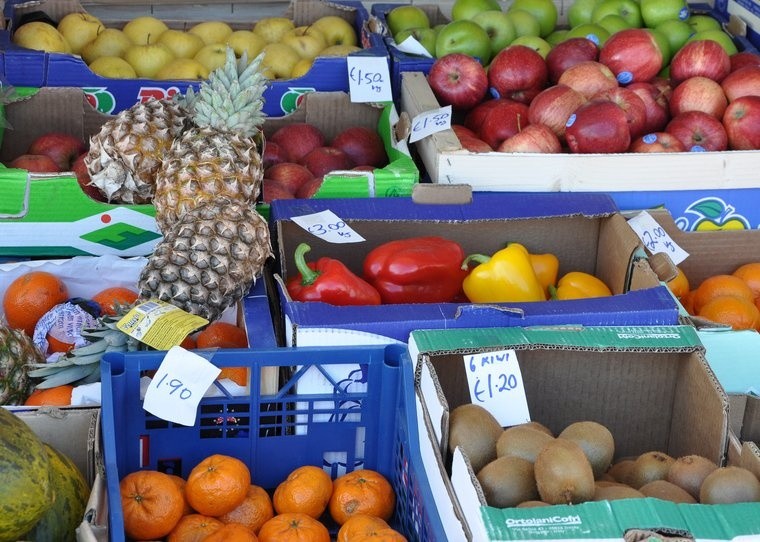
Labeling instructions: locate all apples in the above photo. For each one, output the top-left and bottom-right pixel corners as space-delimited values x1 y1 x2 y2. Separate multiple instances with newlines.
386 0 760 153
260 120 383 203
7 134 108 203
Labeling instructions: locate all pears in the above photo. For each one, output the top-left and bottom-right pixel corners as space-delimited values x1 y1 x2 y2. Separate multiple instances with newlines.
15 12 363 81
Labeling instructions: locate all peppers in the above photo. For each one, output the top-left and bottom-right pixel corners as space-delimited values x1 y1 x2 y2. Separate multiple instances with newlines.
286 236 614 306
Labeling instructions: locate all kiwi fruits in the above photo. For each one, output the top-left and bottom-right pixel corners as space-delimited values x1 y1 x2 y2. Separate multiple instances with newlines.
443 403 760 507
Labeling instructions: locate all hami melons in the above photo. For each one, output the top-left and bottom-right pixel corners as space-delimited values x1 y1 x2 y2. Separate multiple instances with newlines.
0 407 93 542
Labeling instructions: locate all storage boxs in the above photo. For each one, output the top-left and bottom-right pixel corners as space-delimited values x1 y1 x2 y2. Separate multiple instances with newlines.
0 0 760 542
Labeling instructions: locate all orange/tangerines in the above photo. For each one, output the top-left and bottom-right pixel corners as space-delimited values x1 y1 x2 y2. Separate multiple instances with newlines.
122 453 408 542
4 270 249 407
662 262 759 334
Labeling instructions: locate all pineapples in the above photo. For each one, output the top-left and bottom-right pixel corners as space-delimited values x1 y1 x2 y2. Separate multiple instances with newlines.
0 318 42 406
84 46 273 331
